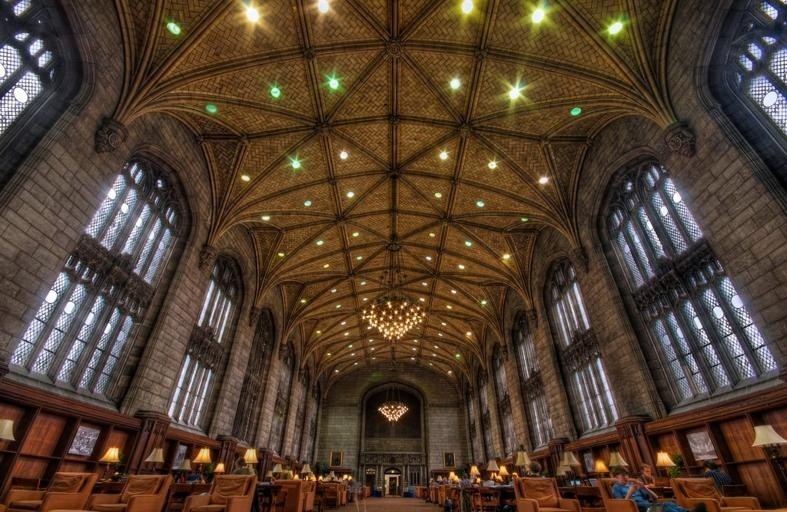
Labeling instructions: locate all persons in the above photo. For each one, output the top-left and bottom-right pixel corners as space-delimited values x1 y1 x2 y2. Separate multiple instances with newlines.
233 456 250 475
501 463 517 484
202 472 208 484
612 465 708 512
203 464 214 483
429 473 472 488
701 459 732 486
635 464 657 489
270 470 357 505
390 479 396 496
497 461 546 512
479 471 494 500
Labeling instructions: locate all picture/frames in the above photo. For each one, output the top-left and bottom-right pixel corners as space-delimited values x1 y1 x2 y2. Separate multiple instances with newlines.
443 450 456 469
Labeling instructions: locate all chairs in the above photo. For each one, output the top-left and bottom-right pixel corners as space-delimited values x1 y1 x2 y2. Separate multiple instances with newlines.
414 473 760 511
0 471 371 512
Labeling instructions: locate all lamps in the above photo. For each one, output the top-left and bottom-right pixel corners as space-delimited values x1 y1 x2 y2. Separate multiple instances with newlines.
0 416 19 442
750 424 787 480
377 342 410 423
360 232 426 340
468 441 629 481
655 450 677 479
99 448 120 479
142 446 352 483
